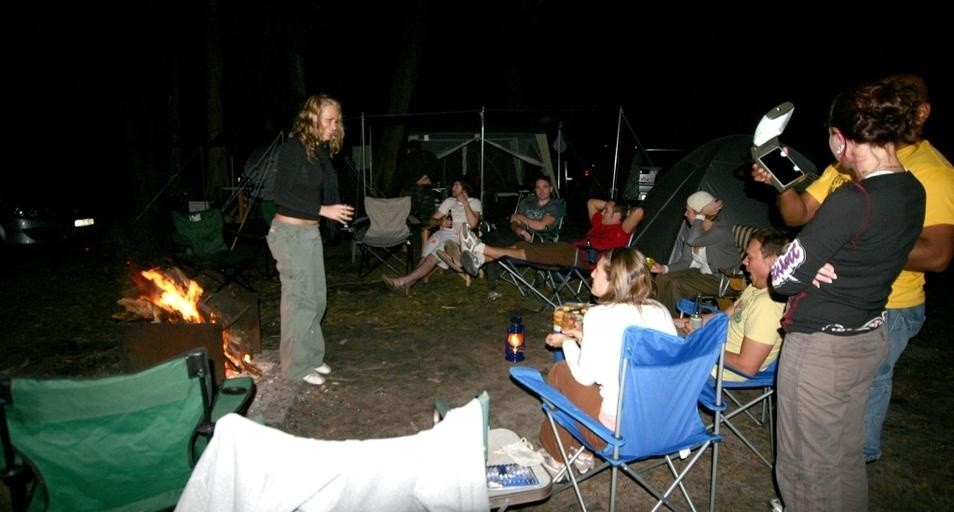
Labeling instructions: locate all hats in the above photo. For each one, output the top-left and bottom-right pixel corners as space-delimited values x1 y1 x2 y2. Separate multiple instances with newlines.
687 191 714 212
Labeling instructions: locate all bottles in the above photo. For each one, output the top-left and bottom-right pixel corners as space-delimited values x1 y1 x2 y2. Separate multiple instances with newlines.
585 237 592 248
447 208 453 231
690 291 706 336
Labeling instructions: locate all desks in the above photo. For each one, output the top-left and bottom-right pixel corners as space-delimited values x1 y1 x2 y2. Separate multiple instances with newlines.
484 426 555 512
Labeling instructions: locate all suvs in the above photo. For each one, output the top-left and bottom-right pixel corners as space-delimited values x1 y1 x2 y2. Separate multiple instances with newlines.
637 148 693 203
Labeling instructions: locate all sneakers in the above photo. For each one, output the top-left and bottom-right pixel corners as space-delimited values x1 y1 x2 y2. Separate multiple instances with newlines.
538 447 594 484
437 222 482 277
303 362 331 385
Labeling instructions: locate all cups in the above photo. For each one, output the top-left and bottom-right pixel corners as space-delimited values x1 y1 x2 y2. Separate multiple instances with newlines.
338 204 356 230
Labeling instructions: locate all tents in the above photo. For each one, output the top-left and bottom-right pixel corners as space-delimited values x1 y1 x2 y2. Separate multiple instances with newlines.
629 134 822 280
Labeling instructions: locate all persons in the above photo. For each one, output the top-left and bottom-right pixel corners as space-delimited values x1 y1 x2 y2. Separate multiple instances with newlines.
672 226 790 384
765 83 928 512
646 187 749 320
262 92 356 388
534 244 681 485
749 67 954 468
457 198 646 279
434 174 565 276
397 139 441 227
379 177 484 294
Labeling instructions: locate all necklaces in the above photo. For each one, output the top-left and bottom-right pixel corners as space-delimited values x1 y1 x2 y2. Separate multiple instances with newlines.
857 164 904 180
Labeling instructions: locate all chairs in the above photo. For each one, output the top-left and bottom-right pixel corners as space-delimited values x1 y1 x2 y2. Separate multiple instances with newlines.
530 225 637 309
667 297 786 470
169 384 495 512
507 310 726 512
498 196 565 296
168 206 256 296
348 186 488 296
0 341 261 512
648 222 770 320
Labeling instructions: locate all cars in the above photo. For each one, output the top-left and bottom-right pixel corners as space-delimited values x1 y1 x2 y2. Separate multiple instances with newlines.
1 171 105 259
